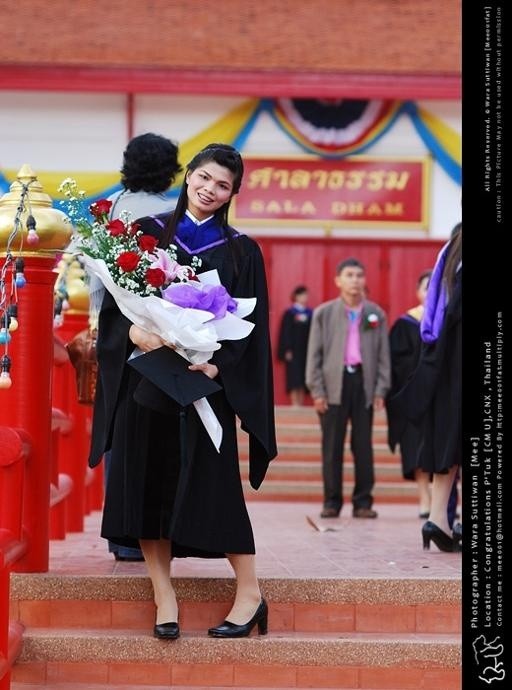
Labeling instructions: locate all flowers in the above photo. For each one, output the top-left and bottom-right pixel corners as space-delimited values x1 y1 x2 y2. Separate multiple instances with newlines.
60 179 256 367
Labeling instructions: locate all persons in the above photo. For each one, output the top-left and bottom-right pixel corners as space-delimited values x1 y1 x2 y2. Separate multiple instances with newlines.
77 129 463 640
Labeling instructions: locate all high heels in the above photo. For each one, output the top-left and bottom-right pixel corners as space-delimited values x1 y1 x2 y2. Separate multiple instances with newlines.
155 606 180 640
418 493 431 519
422 521 461 551
208 598 269 638
453 519 463 553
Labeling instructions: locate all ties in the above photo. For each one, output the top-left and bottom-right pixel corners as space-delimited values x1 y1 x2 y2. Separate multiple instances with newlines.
351 311 359 320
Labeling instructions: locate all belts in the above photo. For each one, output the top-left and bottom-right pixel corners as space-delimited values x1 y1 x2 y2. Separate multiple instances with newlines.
345 364 360 372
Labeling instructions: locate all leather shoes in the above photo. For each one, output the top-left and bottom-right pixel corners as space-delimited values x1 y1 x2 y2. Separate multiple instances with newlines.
320 508 341 518
351 508 378 518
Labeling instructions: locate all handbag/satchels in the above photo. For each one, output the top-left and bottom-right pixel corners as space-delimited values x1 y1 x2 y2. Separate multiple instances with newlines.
65 329 98 402
